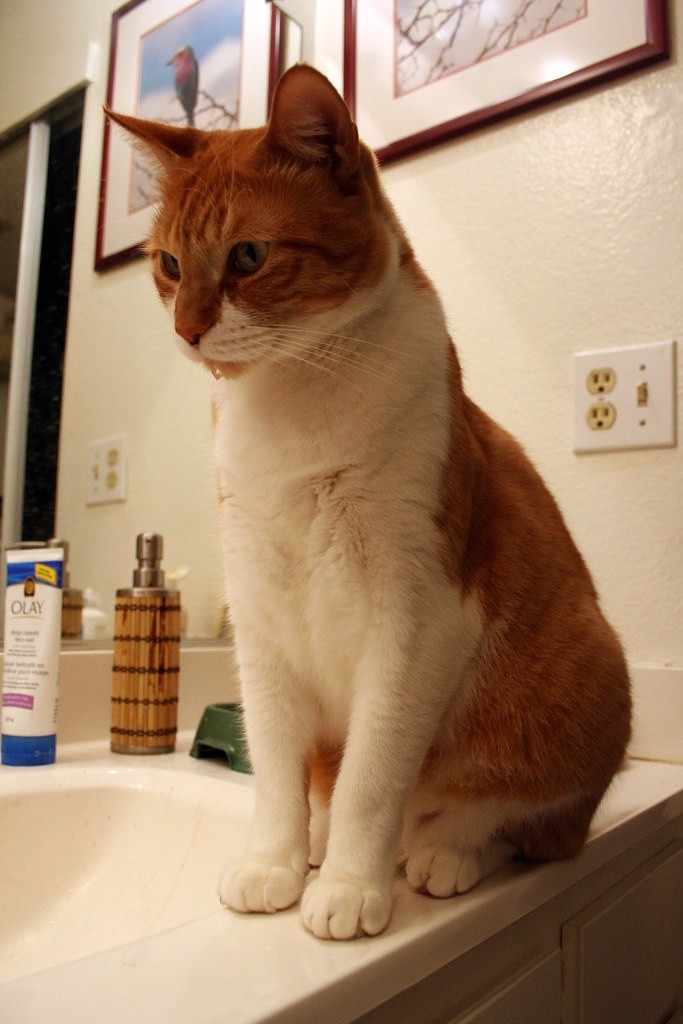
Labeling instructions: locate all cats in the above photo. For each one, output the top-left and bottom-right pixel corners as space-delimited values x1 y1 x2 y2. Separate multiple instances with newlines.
102 61 632 942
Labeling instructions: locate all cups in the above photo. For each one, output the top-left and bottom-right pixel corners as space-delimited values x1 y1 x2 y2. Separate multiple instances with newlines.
187 599 225 640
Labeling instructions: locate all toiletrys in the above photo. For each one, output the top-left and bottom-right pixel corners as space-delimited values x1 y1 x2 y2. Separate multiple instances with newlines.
107 531 183 757
0 547 65 768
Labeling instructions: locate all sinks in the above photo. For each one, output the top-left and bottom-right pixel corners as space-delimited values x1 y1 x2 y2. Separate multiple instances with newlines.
0 751 323 986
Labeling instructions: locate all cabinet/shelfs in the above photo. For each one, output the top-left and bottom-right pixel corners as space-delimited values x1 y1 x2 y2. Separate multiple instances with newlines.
348 811 683 1024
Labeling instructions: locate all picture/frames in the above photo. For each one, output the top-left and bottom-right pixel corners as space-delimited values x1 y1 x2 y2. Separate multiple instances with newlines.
92 0 281 270
342 0 673 169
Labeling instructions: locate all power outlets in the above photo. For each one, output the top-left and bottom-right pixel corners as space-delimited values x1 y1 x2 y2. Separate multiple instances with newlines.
572 338 674 453
85 434 128 506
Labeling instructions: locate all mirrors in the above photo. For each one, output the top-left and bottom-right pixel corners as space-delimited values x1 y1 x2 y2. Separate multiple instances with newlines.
0 0 352 648
